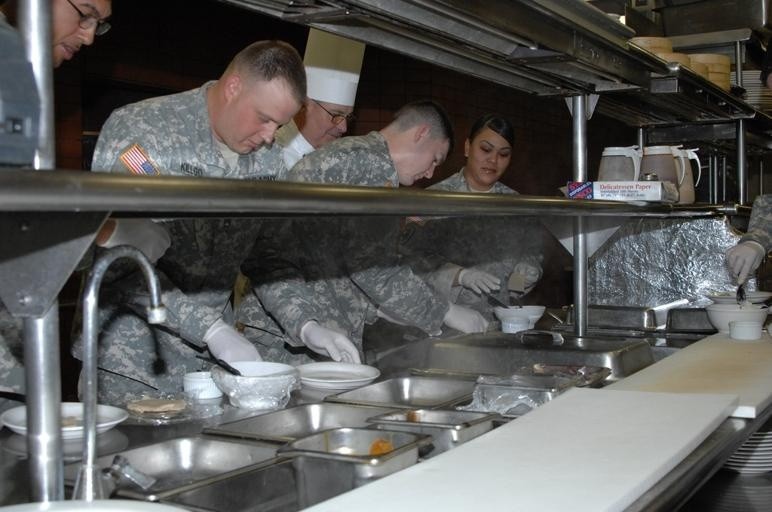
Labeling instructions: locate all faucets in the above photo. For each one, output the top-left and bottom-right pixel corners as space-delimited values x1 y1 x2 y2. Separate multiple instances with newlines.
72 241 168 504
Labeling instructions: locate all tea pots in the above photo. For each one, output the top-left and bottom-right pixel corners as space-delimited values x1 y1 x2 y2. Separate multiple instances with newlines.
597 144 640 182
642 143 686 186
672 147 702 206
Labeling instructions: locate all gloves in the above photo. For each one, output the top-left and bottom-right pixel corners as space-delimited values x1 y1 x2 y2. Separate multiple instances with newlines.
443 301 488 333
458 267 500 294
300 320 361 364
97 219 171 264
514 263 539 288
725 241 765 285
377 310 442 337
202 318 262 361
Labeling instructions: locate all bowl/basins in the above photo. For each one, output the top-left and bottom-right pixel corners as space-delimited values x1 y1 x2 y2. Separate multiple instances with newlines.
493 304 546 331
211 360 301 410
501 318 530 334
704 304 769 335
183 372 225 400
728 321 763 341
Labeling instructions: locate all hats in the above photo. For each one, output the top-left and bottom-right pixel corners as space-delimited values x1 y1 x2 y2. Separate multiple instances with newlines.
303 28 365 107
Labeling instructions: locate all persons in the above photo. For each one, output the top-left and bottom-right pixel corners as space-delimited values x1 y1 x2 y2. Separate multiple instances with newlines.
0 1 173 393
401 111 553 326
268 96 357 175
70 39 362 412
724 193 772 286
234 98 490 365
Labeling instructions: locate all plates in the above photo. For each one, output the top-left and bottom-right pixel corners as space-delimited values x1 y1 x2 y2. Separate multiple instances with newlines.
0 428 129 461
293 361 382 391
710 292 772 304
730 69 772 112
722 427 772 475
714 477 772 512
0 399 131 441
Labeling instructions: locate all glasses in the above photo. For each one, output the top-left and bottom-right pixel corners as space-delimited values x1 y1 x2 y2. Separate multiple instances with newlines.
314 102 359 124
68 0 111 36
